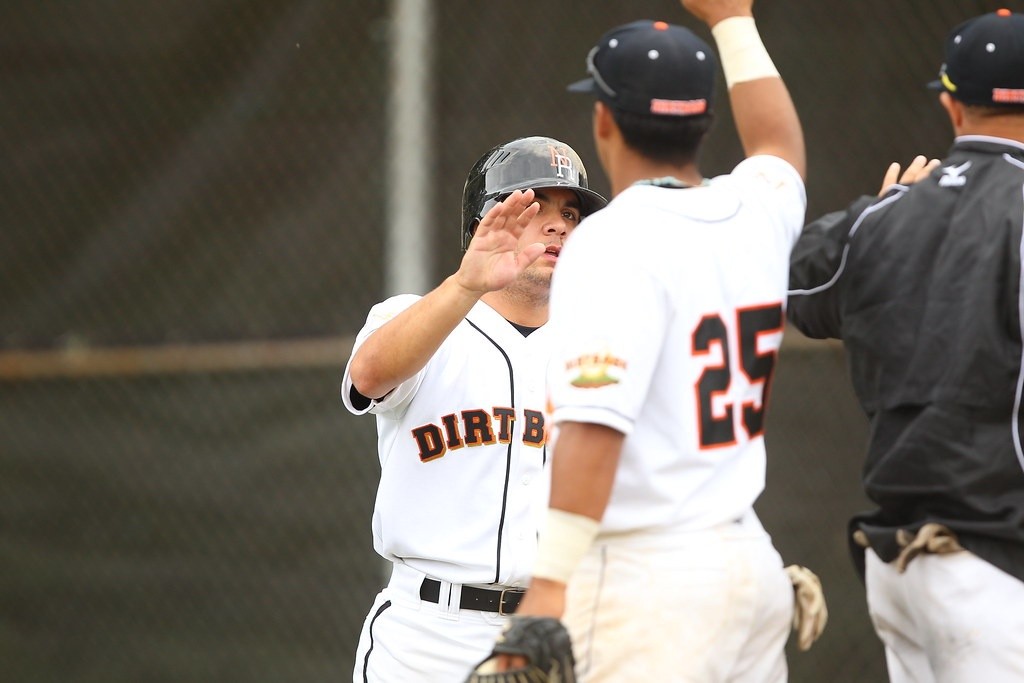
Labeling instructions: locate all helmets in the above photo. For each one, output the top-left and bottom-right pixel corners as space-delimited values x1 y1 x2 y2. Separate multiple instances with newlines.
461 135 607 252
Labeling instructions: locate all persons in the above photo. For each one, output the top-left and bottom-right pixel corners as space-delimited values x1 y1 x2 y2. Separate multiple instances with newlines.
786 9 1024 683
341 136 609 683
468 0 806 683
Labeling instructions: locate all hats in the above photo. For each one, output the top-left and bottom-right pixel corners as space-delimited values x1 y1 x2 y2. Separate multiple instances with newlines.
566 19 716 117
925 8 1024 107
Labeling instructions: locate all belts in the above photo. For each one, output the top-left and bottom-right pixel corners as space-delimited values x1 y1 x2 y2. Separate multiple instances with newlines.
419 577 525 616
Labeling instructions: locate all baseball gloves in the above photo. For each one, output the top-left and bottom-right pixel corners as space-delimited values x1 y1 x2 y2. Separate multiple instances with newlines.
466 615 577 683
